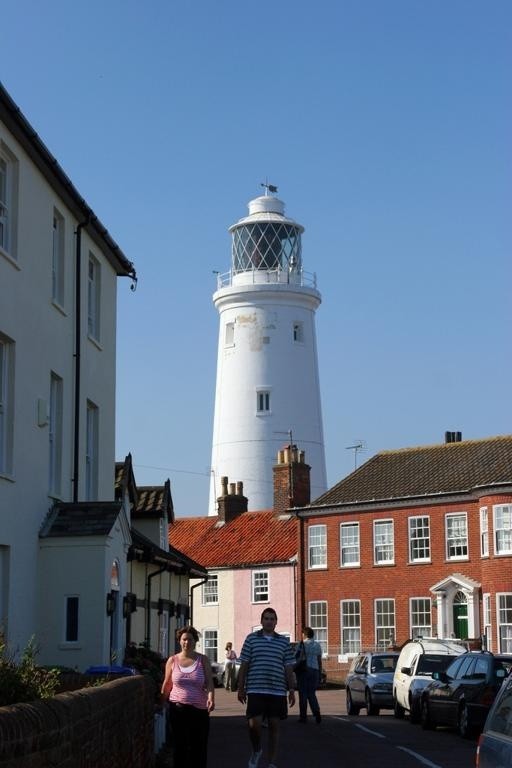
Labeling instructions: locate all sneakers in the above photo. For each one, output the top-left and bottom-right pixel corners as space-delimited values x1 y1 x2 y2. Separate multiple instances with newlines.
247 748 264 767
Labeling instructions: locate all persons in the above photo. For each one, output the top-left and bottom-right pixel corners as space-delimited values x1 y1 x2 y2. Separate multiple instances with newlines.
225 642 237 692
237 608 296 767
296 627 322 723
160 626 215 767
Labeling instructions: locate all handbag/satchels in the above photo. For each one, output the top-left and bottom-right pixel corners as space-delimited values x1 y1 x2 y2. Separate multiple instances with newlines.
294 640 307 663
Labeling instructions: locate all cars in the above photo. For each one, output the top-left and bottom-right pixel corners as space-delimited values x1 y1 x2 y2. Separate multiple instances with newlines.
345 636 512 768
211 660 326 690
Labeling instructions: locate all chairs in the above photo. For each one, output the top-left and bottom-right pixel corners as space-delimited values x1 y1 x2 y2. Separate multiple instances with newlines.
373 659 384 673
